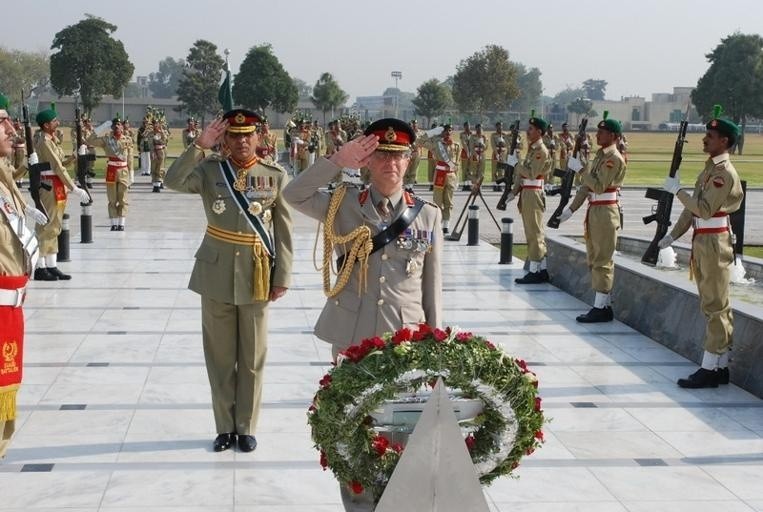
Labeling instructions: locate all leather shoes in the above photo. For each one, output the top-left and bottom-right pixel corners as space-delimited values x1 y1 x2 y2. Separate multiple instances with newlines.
111 225 124 231
238 435 256 452
678 367 729 388
576 305 613 322
34 267 71 281
213 433 236 452
515 268 549 284
152 187 160 192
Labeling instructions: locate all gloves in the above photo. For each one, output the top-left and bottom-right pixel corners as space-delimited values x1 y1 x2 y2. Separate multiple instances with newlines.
567 151 584 174
23 152 39 170
661 169 683 195
505 193 515 205
507 151 518 167
426 126 444 138
556 208 573 223
657 233 674 250
127 171 135 187
93 121 113 137
24 204 48 226
72 187 90 204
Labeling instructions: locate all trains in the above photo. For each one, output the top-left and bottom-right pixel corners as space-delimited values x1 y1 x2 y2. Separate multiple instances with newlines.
658 122 762 133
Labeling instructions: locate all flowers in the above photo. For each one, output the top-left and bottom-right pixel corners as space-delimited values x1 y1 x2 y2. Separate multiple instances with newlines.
305 321 546 502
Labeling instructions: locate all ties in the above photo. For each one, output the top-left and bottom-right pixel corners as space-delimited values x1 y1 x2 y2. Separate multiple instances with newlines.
382 198 389 213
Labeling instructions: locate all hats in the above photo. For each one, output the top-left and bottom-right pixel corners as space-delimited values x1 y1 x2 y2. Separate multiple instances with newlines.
363 118 417 153
0 92 9 111
529 118 549 131
598 119 622 134
113 117 121 123
706 118 740 137
222 109 264 134
36 109 58 126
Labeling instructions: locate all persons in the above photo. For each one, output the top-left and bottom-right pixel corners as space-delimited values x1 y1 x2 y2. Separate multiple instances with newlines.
73 108 96 191
182 117 200 148
557 118 626 323
658 116 734 390
32 107 90 282
10 118 27 190
502 119 552 286
88 118 136 232
164 110 294 453
404 120 638 235
280 117 444 364
0 90 25 458
258 113 373 172
136 104 171 193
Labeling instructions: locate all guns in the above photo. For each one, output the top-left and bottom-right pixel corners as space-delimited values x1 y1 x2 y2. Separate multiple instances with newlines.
76 96 95 206
496 120 520 210
641 103 691 266
546 118 588 228
22 90 52 226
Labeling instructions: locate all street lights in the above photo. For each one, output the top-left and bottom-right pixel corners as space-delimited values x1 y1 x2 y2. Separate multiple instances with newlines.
390 70 403 112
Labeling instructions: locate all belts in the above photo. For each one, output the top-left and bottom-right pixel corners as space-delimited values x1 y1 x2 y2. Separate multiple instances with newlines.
522 178 542 188
474 147 483 152
435 165 454 171
154 145 163 149
107 160 128 167
257 150 271 155
0 285 27 308
495 147 506 151
588 192 617 202
41 170 56 176
691 216 729 230
